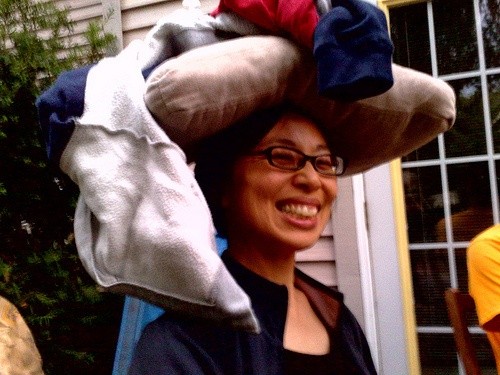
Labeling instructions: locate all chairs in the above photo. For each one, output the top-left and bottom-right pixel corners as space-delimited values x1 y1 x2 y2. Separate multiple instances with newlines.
446 289 500 375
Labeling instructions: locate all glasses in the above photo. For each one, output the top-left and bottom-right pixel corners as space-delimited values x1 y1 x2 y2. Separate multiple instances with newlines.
236 145 345 178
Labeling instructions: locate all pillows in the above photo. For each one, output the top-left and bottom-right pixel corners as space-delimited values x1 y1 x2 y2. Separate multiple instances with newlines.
142 35 457 178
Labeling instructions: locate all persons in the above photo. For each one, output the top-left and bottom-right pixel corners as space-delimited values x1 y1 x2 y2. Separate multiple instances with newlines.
126 103 381 375
437 167 500 326
